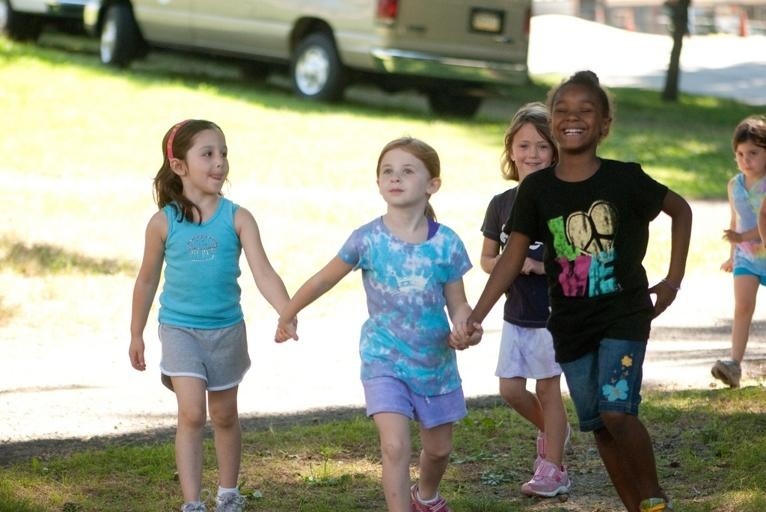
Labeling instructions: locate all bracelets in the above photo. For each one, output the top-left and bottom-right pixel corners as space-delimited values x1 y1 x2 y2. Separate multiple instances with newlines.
660 276 680 293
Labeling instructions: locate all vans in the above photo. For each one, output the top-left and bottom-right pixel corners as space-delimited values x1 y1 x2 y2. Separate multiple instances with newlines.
67 0 535 121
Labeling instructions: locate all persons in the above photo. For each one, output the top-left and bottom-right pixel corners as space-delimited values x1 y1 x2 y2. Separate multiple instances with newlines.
713 112 766 386
275 135 484 512
462 70 692 511
477 100 582 499
127 120 295 511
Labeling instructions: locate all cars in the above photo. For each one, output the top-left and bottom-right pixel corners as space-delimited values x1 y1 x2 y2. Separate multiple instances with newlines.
0 0 54 43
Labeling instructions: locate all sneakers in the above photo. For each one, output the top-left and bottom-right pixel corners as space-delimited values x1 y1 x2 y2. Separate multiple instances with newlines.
521 459 571 496
533 422 570 472
214 490 247 512
711 359 741 387
410 479 454 512
639 498 674 512
180 501 206 512
